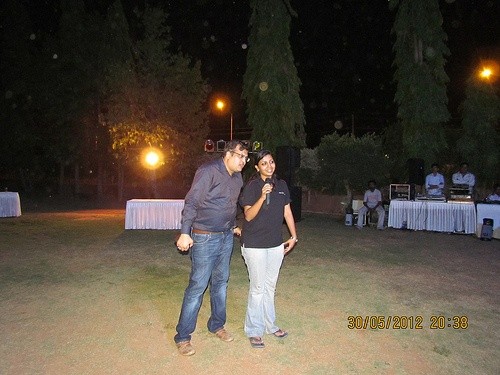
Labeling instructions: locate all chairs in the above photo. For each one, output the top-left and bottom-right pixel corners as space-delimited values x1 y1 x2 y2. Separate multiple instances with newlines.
352 200 366 225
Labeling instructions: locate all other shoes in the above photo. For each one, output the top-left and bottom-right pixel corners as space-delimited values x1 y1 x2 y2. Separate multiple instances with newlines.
214 327 234 342
176 341 196 356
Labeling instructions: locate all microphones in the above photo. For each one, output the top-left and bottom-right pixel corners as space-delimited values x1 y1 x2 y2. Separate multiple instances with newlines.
264 178 272 205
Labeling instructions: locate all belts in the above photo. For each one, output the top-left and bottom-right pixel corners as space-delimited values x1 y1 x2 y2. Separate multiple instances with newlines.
191 228 225 234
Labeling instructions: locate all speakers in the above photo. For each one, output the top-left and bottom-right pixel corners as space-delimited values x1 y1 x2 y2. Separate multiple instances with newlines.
275 145 301 169
408 158 425 185
283 186 301 223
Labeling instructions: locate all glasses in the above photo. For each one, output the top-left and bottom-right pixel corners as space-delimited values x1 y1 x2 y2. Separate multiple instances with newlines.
230 151 250 163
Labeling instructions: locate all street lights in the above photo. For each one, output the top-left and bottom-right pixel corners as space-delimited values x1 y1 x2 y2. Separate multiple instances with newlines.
217 101 233 141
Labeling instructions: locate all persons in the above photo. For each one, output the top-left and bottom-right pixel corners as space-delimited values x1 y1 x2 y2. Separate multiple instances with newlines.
425 164 444 196
174 142 250 355
453 163 476 195
485 184 500 201
239 150 298 348
355 181 386 231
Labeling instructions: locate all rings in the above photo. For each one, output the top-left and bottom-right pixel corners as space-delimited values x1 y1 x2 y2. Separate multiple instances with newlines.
184 248 187 249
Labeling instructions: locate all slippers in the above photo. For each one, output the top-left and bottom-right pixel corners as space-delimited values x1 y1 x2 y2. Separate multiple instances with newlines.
273 329 287 338
249 337 265 348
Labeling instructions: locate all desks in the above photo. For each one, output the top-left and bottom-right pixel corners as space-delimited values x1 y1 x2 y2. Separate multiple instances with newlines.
0 192 21 217
474 199 500 239
388 199 477 234
125 199 185 230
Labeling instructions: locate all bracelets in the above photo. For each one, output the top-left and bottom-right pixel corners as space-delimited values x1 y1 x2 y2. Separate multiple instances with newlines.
233 226 238 229
291 237 298 242
